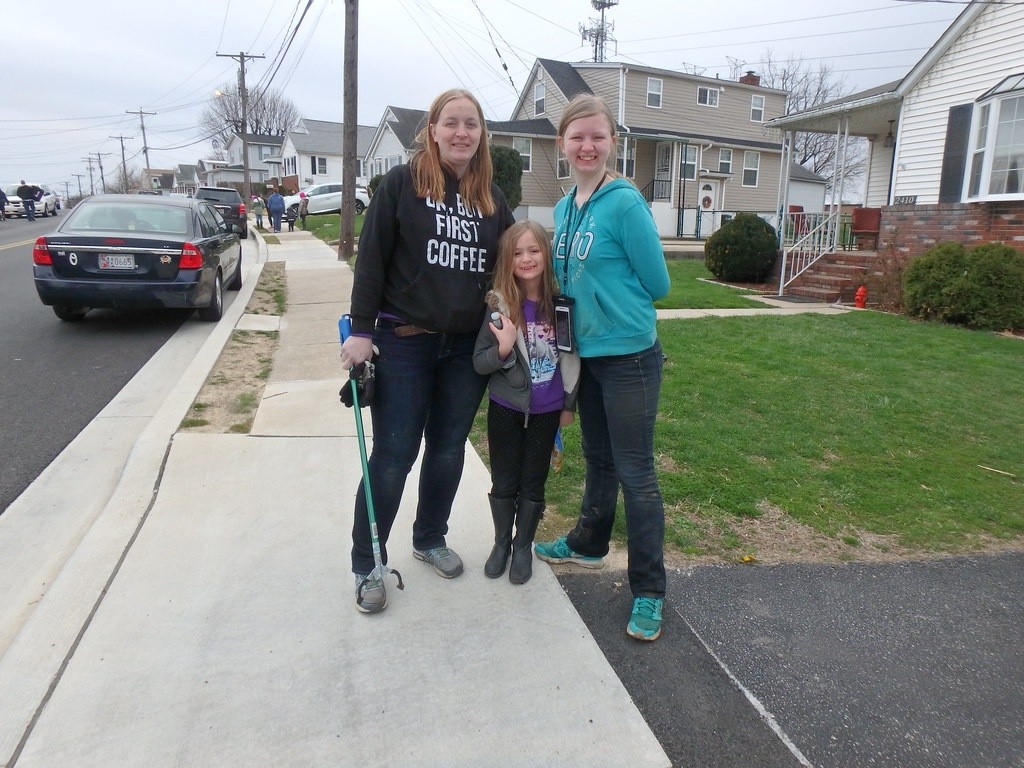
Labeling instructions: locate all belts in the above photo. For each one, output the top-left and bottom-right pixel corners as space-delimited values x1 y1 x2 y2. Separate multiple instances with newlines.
395 323 439 338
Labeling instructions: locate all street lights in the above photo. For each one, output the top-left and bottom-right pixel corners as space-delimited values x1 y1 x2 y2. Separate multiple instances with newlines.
215 90 251 213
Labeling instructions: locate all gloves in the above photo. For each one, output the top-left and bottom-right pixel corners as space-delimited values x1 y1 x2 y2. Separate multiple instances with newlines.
339 343 379 409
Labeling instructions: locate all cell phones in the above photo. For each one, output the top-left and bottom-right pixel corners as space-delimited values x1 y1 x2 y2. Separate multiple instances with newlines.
554 305 572 353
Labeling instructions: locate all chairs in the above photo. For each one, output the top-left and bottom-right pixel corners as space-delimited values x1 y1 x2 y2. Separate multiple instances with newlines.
787 205 833 254
848 207 881 253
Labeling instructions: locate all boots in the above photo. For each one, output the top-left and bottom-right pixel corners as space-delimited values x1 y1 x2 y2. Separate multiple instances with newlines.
257 221 259 228
483 493 516 579
260 221 263 229
509 496 546 584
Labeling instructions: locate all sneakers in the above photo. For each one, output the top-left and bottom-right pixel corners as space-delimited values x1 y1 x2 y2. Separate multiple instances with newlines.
534 535 606 569
413 545 463 579
626 598 665 642
354 573 388 613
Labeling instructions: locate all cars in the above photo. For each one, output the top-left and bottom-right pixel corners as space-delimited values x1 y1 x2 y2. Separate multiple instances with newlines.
4 184 61 218
33 194 244 321
282 183 370 218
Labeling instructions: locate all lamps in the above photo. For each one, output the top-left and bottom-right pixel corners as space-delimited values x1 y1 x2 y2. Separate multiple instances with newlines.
883 120 897 148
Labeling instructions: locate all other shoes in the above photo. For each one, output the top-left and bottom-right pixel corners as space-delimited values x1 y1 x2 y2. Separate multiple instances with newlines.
275 229 280 233
30 215 35 221
2 216 5 221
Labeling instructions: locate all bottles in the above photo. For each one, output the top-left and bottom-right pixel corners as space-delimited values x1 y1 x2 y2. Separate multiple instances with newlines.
490 311 517 369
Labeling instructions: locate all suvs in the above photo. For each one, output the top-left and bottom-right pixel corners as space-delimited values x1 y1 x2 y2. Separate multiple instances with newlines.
193 187 247 239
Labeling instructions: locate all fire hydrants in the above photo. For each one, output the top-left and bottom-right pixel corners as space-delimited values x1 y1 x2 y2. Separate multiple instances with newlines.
855 285 868 307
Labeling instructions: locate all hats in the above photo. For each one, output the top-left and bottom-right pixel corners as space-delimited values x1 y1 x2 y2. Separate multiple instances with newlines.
300 192 305 198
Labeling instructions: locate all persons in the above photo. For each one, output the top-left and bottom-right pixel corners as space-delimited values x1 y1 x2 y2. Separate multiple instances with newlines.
340 89 517 613
17 180 36 222
253 194 275 229
472 218 581 585
284 206 298 232
268 188 285 233
534 94 670 641
0 189 9 221
298 192 309 231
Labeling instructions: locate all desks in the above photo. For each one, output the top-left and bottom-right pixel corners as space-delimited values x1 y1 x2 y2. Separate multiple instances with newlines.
818 219 855 252
